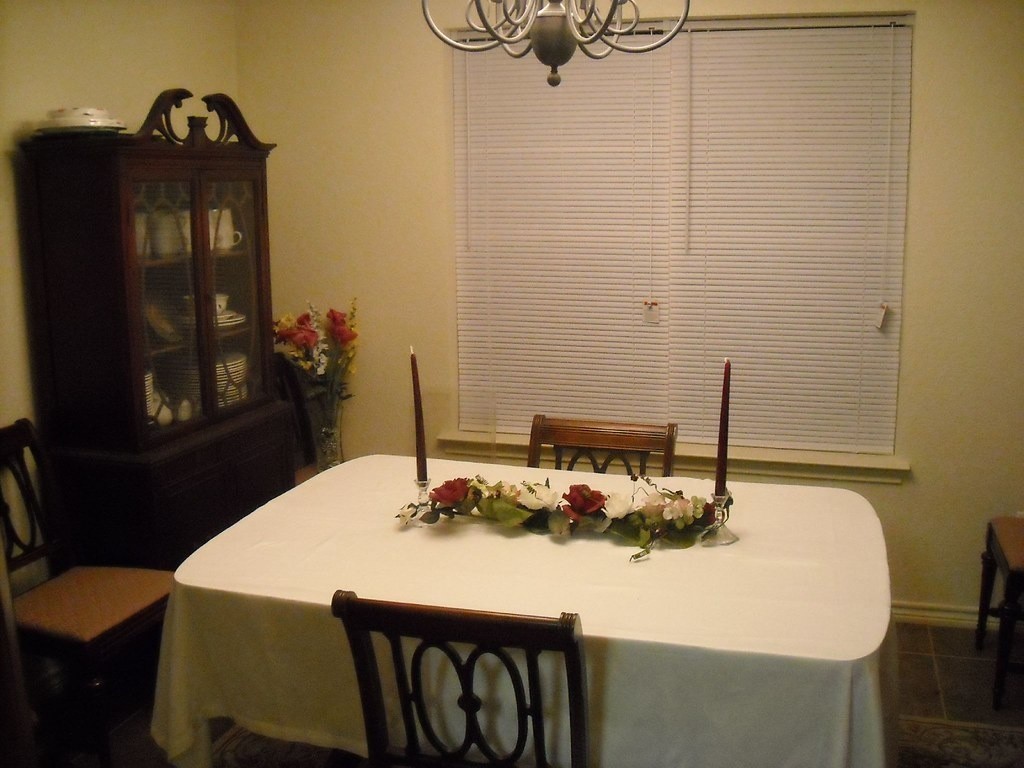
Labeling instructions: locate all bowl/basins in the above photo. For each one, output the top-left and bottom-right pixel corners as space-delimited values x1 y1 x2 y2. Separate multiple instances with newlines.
177 294 229 316
130 211 242 258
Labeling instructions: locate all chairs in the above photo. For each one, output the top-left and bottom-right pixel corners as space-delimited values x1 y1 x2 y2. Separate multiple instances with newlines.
0 418 174 768
331 589 589 768
527 414 678 477
975 516 1024 711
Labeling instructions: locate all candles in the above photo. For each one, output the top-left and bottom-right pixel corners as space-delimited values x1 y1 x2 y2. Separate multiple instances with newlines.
410 346 427 482
714 357 731 497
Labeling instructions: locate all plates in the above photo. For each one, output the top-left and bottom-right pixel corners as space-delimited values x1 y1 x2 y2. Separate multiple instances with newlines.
176 313 247 332
143 349 251 426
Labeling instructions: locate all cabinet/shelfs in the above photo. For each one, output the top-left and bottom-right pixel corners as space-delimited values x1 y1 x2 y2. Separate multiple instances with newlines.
19 88 295 570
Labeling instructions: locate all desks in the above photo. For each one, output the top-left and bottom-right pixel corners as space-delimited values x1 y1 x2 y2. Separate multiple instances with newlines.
150 453 900 768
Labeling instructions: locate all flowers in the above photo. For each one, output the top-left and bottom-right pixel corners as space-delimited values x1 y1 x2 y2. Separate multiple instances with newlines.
272 297 359 475
394 474 733 563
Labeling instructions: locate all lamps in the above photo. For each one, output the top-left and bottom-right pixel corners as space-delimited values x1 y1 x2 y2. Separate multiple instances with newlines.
421 0 690 87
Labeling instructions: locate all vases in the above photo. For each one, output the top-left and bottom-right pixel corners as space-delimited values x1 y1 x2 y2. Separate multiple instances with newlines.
301 379 342 471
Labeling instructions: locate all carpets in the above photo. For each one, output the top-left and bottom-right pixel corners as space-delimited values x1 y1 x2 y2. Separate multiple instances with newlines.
206 713 1024 768
